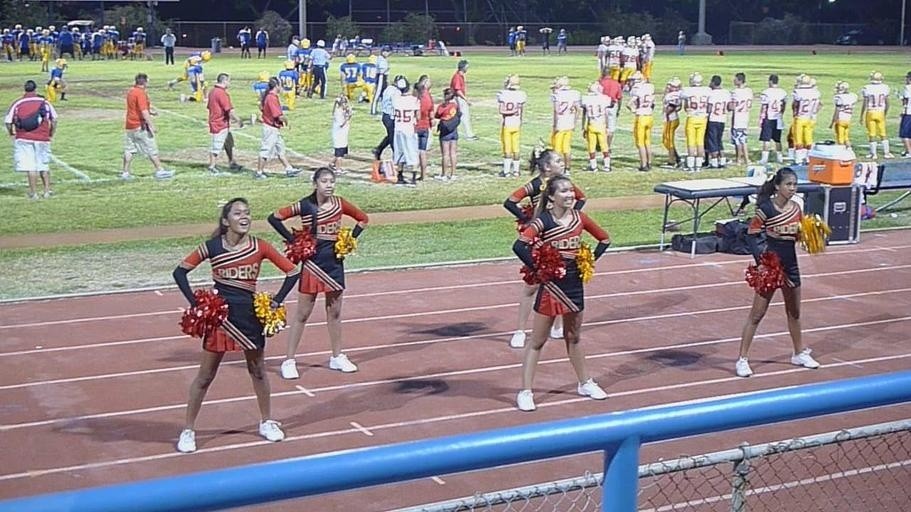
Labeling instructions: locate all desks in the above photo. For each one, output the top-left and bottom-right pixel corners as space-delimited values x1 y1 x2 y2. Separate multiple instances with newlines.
654 176 829 261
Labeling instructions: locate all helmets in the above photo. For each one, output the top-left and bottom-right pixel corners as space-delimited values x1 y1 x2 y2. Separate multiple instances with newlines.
797 74 811 85
259 71 269 81
870 71 883 84
588 80 604 93
836 81 849 94
56 59 67 69
554 75 568 88
346 54 356 63
506 73 520 90
188 57 197 65
627 70 644 83
292 36 325 49
201 51 211 61
689 72 703 86
369 55 377 64
285 60 295 70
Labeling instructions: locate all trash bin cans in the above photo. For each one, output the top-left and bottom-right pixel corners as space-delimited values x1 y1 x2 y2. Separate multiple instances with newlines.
211 38 222 54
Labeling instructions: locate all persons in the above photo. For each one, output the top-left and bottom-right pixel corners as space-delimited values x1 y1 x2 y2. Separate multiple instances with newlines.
502 149 587 349
206 72 243 173
829 70 910 160
495 74 526 177
49 59 68 100
597 34 655 90
4 79 59 200
0 24 147 71
168 50 212 101
236 26 269 59
678 30 686 54
118 73 177 179
250 35 331 124
509 26 567 56
171 197 301 453
161 28 176 66
756 73 823 164
256 77 304 179
372 60 478 184
658 73 754 171
736 167 820 378
267 166 368 380
552 71 654 173
509 176 611 412
329 94 351 173
332 33 390 115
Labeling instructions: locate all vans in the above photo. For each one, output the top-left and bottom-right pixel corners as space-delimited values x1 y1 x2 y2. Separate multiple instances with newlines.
67 20 95 33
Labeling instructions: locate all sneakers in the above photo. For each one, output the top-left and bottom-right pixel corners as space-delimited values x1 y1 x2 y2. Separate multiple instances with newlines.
251 114 257 125
281 359 299 379
229 163 241 169
577 378 607 399
583 166 612 172
736 356 753 377
517 390 536 411
286 168 303 177
178 429 196 452
551 325 564 338
259 419 284 441
372 147 381 159
660 155 726 171
511 330 526 348
209 165 219 174
467 136 478 141
791 348 820 368
156 171 172 177
330 354 357 372
901 151 911 158
865 153 878 160
499 171 520 177
395 172 456 187
884 153 894 158
255 172 268 178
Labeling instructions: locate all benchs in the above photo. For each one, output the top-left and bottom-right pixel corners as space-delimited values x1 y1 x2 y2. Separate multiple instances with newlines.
862 161 910 214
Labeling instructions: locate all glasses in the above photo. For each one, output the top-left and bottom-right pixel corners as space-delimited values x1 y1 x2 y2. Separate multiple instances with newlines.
666 77 681 92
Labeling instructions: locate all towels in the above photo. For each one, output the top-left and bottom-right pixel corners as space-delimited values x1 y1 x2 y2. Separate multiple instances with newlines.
862 163 880 190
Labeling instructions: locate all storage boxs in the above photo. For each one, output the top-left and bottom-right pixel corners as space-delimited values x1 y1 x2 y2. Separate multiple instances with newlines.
808 142 856 185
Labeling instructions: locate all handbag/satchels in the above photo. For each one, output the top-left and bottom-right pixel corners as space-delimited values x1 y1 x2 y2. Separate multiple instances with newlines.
14 102 48 131
441 114 460 134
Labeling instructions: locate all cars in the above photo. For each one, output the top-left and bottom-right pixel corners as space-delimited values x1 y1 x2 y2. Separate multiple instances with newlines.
836 24 890 47
